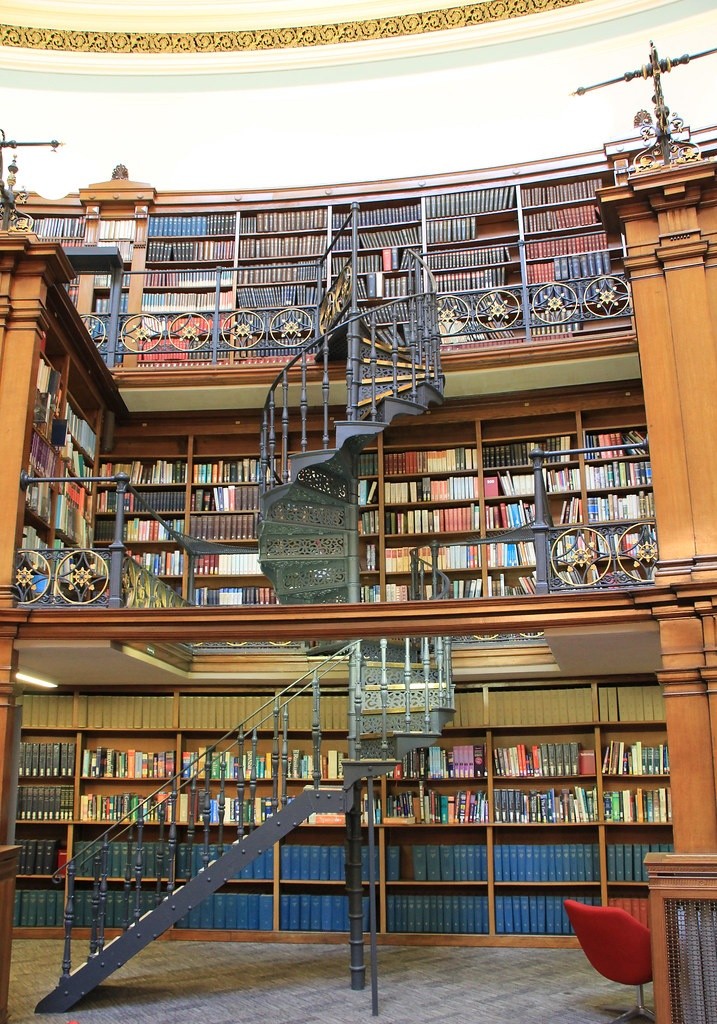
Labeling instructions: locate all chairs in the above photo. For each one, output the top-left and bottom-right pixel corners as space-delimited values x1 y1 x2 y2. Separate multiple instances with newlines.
564 900 654 1024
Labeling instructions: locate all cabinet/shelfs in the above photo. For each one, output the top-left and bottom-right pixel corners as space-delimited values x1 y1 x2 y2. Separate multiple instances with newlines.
92 423 657 602
12 676 673 948
19 309 107 603
87 215 137 368
0 205 87 308
136 170 638 368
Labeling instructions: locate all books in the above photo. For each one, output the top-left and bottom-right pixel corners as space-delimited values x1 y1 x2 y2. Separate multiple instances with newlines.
23 358 658 606
31 178 619 365
12 742 673 936
